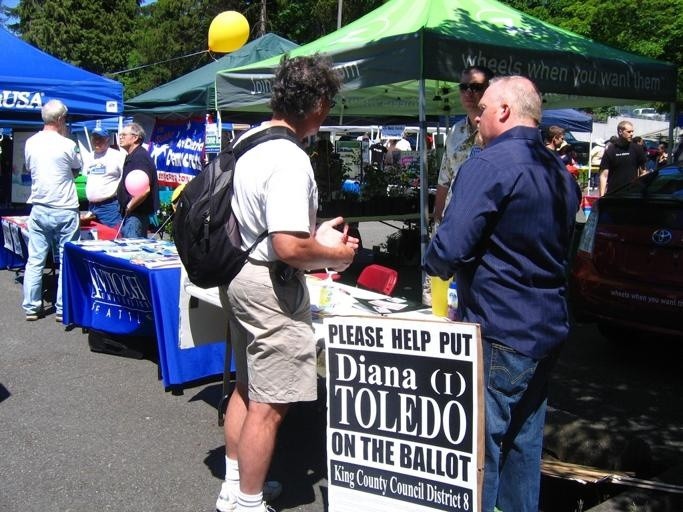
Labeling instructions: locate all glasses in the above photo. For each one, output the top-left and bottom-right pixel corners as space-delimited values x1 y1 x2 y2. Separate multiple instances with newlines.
121 132 139 137
458 82 487 92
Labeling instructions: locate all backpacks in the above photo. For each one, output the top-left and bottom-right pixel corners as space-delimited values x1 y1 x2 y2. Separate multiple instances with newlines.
172 125 308 288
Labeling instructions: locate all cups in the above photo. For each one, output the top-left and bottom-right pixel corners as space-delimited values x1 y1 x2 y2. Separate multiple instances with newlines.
430 275 454 318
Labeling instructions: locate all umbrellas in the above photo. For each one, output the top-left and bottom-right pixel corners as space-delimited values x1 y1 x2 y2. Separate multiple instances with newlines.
1 24 124 124
215 1 676 301
541 108 593 133
123 33 302 113
0 116 134 132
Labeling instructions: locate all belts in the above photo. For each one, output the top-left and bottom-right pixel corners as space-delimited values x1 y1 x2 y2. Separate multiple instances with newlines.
91 195 117 206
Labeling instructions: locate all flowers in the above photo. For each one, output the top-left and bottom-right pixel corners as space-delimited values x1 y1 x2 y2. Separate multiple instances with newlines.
399 163 420 213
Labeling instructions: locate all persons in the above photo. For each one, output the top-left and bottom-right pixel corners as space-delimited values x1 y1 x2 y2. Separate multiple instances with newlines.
82 127 124 228
599 121 646 196
368 138 413 170
117 123 160 238
434 65 495 225
590 137 605 190
215 53 359 512
423 76 582 401
634 134 670 178
545 124 574 168
20 99 83 322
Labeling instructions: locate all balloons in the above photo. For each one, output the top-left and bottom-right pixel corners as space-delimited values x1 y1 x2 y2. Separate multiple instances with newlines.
171 184 191 212
124 170 150 198
208 11 250 52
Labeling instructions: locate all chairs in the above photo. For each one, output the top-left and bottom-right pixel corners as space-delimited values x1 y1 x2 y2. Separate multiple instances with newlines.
308 272 340 281
357 264 398 296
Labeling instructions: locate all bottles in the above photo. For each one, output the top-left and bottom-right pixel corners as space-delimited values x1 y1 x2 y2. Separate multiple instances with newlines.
320 279 334 315
447 274 464 322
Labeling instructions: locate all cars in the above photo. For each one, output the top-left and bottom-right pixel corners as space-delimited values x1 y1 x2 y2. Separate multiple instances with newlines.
641 140 657 161
633 108 660 120
577 161 683 350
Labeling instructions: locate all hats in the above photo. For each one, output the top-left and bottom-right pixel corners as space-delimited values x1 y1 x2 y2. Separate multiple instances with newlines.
91 127 111 138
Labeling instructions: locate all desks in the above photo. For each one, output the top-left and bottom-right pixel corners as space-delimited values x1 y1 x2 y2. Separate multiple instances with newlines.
3 216 121 292
63 238 234 387
189 283 453 431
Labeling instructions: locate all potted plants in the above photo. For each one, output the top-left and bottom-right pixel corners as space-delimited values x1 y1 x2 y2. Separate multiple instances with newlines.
388 186 399 211
360 160 391 215
416 149 441 213
332 188 359 217
308 141 352 218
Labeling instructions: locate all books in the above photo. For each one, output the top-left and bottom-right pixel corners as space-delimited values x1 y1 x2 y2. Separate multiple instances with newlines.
116 237 179 257
143 257 182 270
105 245 149 260
352 297 430 316
70 239 118 246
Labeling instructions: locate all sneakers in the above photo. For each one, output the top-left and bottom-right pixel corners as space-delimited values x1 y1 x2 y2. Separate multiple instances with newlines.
26 308 47 320
235 500 277 512
56 313 64 323
216 481 242 512
